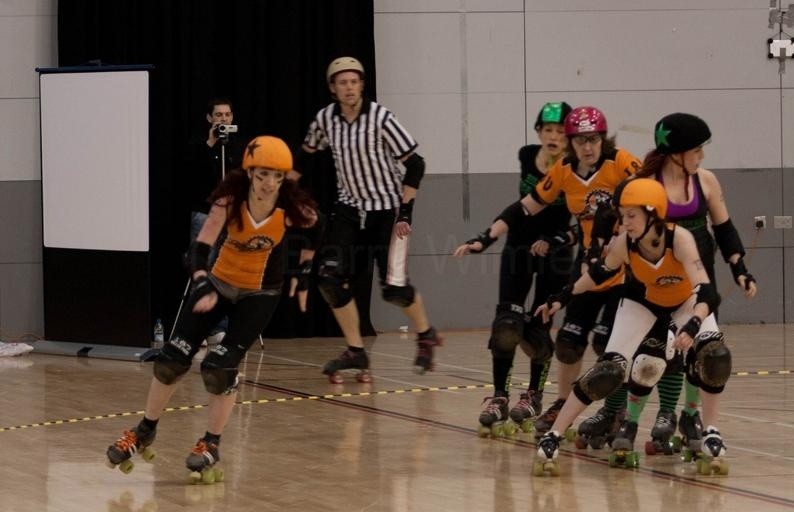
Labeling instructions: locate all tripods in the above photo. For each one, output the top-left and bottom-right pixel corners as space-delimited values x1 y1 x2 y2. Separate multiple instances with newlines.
164 198 265 352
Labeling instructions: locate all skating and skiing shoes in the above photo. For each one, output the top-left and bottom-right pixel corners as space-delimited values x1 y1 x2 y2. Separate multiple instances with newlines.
186 438 225 484
106 423 157 473
413 327 443 374
323 346 370 383
478 388 729 477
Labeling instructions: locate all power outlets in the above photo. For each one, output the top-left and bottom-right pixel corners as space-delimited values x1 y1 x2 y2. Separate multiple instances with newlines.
755 216 766 230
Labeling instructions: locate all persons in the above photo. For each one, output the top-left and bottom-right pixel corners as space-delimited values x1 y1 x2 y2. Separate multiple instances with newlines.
107 136 322 471
301 56 443 376
187 101 251 350
581 113 755 467
477 103 575 438
454 105 643 450
533 180 732 475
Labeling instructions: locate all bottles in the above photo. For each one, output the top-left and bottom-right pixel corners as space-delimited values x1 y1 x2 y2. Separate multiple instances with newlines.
152 315 163 350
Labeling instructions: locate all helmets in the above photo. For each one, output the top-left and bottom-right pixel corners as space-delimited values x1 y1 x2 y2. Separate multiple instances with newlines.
536 101 712 220
327 57 366 83
242 136 294 170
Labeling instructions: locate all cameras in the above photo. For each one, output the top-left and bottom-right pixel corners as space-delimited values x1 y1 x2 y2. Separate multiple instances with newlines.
216 124 237 138
